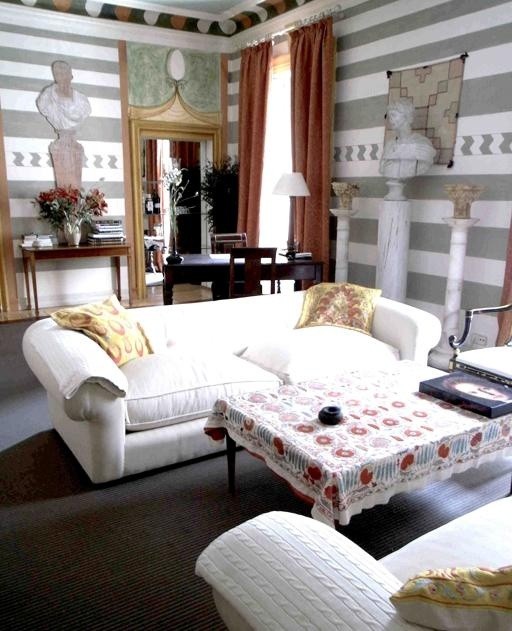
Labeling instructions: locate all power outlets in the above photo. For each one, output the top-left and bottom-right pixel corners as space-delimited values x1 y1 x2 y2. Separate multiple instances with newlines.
474 335 487 345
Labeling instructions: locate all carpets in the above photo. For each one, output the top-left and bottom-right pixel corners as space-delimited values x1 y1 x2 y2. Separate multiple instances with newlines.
0 430 512 631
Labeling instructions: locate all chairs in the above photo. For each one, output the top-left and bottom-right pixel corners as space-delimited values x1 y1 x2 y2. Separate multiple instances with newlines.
228 247 277 299
212 234 247 254
449 304 512 389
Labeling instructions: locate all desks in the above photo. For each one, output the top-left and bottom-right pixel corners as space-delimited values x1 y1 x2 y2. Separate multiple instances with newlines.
162 254 324 305
19 244 132 315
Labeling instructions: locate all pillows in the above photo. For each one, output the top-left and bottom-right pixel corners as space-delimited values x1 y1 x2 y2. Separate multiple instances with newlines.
294 282 383 337
389 565 512 631
51 295 155 367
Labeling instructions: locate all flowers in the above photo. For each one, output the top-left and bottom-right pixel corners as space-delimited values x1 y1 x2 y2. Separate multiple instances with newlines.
164 168 199 253
29 185 109 235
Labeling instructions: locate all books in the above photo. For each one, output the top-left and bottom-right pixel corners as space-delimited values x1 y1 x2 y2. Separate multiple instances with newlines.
85 217 126 247
417 368 512 421
19 232 55 250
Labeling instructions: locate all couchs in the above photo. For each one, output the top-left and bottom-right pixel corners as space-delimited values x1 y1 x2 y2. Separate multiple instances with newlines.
22 281 442 484
195 496 512 631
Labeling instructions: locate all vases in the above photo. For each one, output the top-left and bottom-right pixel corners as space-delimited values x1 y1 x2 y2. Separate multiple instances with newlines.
64 219 81 246
166 254 184 265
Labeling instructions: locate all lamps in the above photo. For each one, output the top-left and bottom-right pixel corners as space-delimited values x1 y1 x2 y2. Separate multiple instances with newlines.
273 172 311 261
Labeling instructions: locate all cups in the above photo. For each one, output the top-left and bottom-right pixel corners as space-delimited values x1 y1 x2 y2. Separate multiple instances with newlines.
319 405 343 425
21 234 60 247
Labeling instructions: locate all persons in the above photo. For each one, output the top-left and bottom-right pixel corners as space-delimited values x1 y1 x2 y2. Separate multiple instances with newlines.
37 61 92 132
441 374 511 406
377 95 438 181
